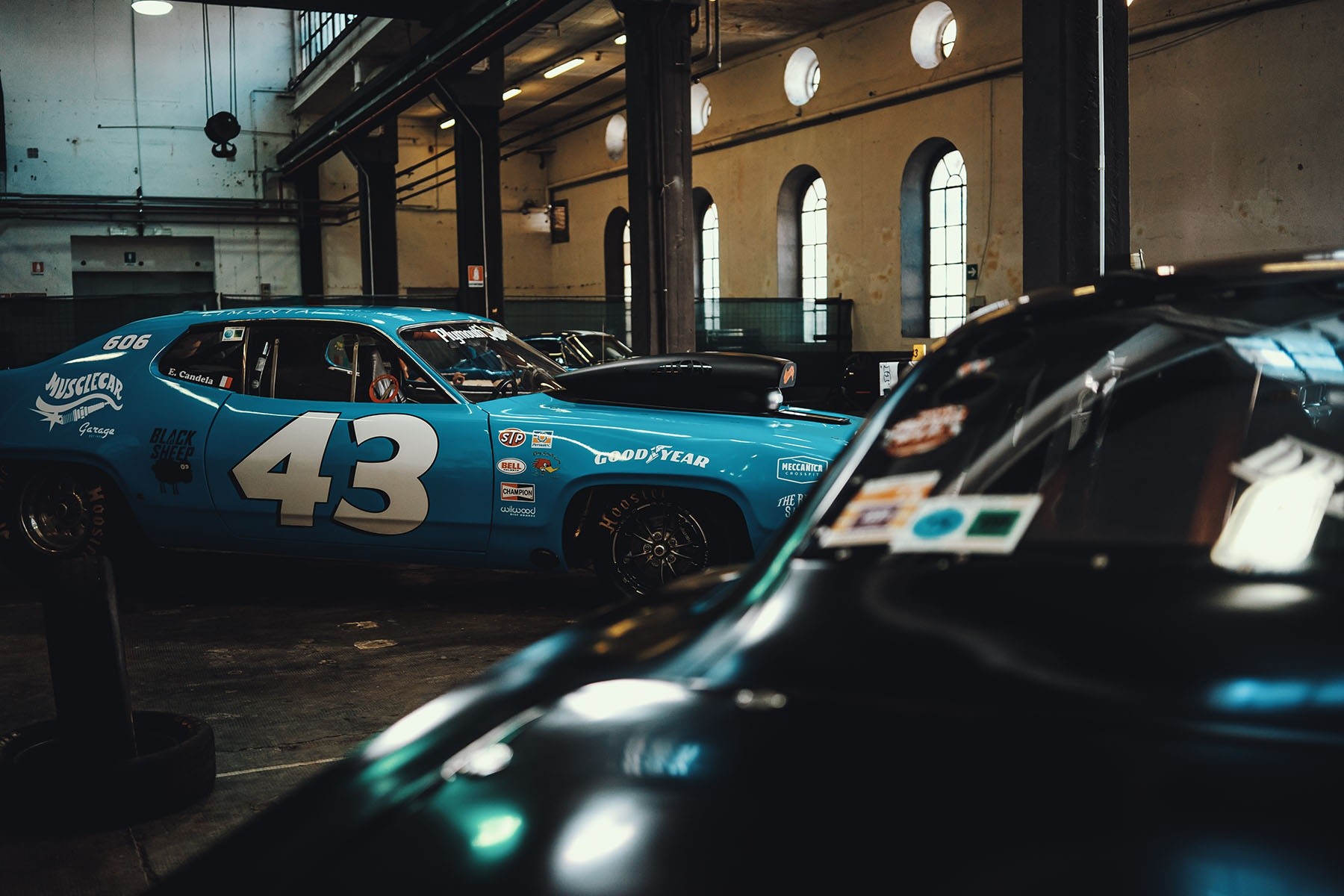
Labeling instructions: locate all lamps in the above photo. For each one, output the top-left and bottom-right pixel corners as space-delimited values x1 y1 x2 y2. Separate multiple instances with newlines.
131 1 173 15
543 57 585 79
503 87 522 100
440 119 454 129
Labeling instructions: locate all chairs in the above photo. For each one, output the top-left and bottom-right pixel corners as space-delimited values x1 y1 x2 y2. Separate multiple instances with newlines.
358 345 408 404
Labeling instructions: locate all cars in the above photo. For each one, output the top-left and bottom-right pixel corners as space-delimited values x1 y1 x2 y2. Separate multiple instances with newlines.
147 243 1344 896
0 304 873 629
